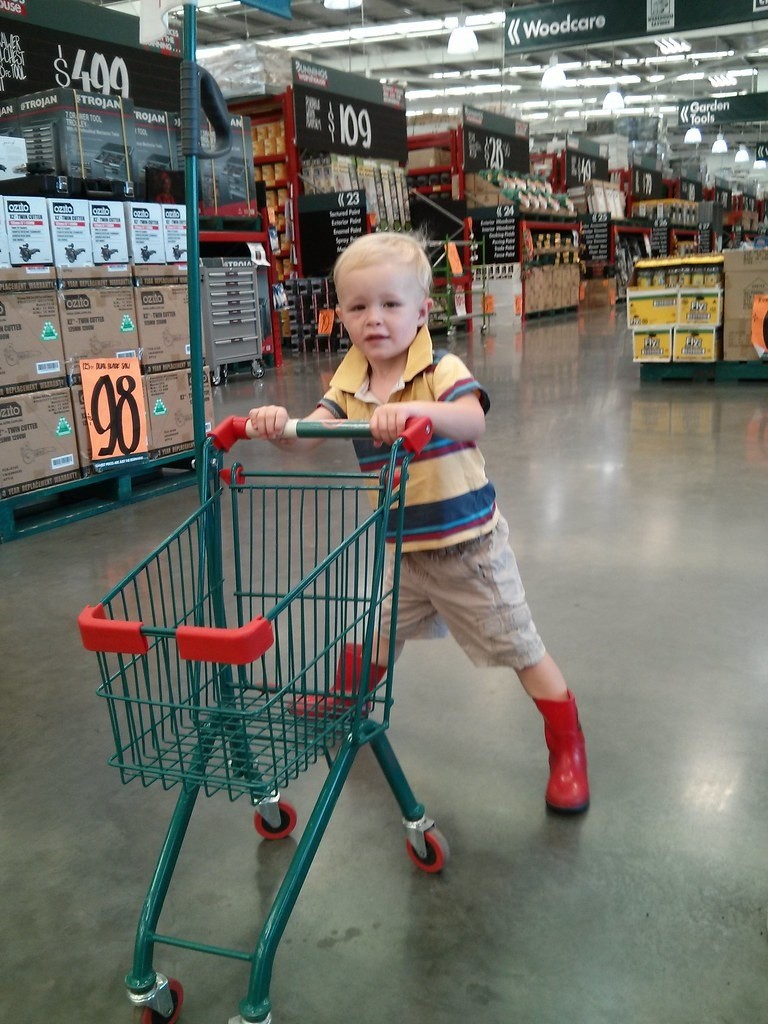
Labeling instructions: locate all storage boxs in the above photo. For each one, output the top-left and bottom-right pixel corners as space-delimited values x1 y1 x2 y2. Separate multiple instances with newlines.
467 169 768 364
285 277 355 352
405 148 450 171
0 87 255 498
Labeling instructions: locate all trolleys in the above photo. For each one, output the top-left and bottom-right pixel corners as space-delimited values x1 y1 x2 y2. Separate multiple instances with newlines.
76 414 456 1024
423 233 496 340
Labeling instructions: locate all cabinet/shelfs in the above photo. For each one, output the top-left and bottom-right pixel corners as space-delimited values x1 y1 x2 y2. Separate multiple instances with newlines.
228 87 409 358
465 208 582 320
404 125 527 329
529 151 768 306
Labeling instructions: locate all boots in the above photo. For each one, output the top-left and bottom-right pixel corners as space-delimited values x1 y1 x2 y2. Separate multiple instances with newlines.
530 690 592 812
287 642 387 718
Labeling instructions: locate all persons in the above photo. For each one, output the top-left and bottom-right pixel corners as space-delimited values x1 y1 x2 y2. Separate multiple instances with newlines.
246 232 590 817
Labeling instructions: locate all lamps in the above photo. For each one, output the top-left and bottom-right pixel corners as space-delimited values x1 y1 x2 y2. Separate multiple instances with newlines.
712 124 728 153
684 58 702 145
734 143 749 162
446 0 479 54
603 56 625 110
752 160 766 169
541 51 566 86
